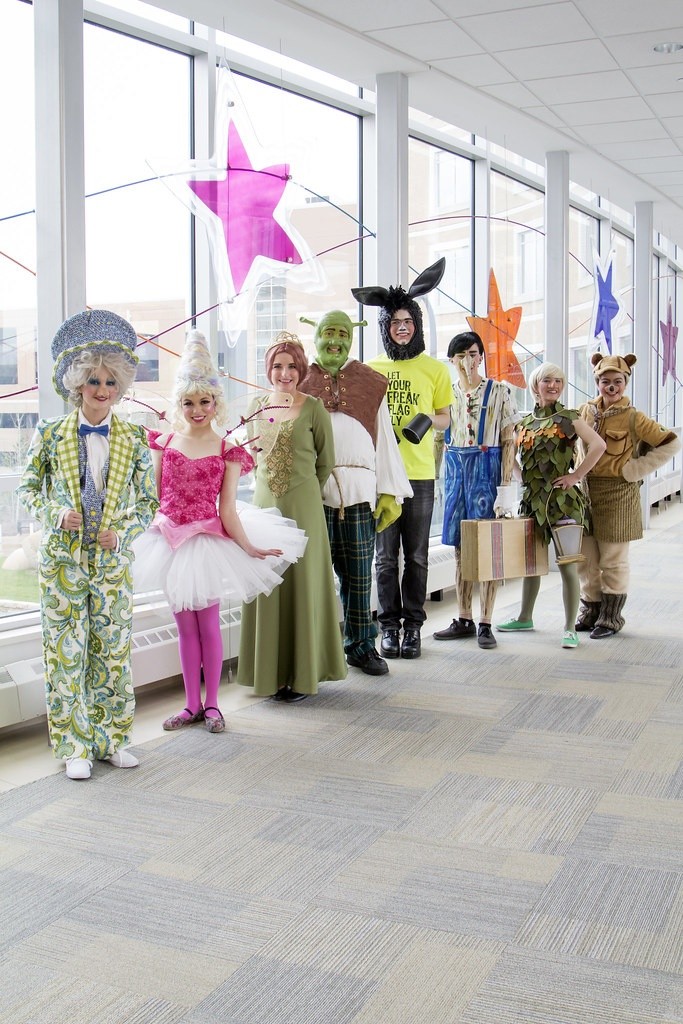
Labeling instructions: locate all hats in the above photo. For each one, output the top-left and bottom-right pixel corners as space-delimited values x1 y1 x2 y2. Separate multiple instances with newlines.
50 309 139 402
590 353 642 384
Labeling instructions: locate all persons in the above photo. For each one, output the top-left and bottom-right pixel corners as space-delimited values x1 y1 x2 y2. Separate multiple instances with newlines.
433 332 523 648
133 329 308 732
350 257 457 659
15 310 160 778
295 311 414 676
236 331 348 703
575 353 682 639
495 362 607 649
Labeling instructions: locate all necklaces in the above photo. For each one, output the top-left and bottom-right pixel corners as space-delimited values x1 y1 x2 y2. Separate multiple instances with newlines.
532 409 566 421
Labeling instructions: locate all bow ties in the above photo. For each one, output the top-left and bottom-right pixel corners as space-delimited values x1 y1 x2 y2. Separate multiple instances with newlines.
79 424 112 438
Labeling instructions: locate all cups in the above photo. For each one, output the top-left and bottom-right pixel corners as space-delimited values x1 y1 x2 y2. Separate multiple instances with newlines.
402 413 432 444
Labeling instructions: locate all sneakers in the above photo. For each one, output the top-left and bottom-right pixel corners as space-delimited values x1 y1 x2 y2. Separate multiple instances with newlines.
477 625 497 649
561 630 579 647
66 757 92 779
433 619 476 640
347 640 389 676
108 749 139 768
495 618 534 632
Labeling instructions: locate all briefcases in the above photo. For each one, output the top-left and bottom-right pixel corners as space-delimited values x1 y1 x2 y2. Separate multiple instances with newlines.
461 506 549 581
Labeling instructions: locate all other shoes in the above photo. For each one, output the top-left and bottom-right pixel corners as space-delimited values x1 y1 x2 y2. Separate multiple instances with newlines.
163 701 205 730
271 687 306 702
204 707 225 733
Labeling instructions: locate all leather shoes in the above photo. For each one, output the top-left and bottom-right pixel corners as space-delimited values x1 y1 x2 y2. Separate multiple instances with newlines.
401 630 421 658
380 629 401 659
590 626 614 639
576 622 590 631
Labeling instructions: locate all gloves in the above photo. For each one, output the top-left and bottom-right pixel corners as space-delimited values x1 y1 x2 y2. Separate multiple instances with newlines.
373 494 403 532
492 484 518 515
